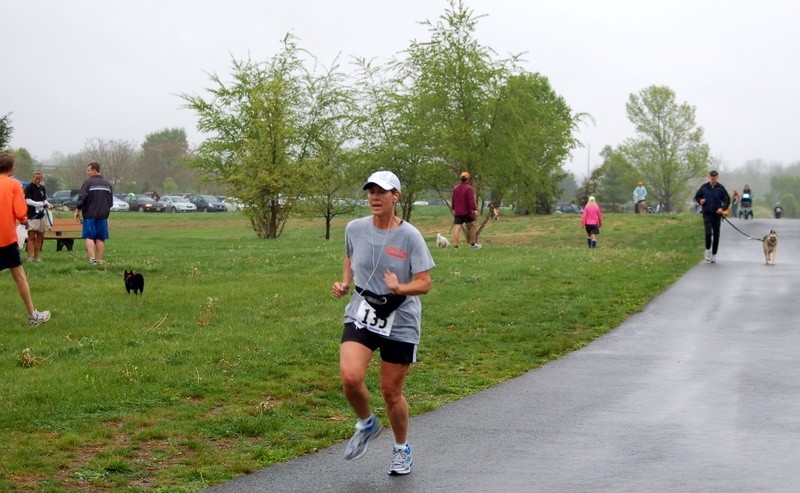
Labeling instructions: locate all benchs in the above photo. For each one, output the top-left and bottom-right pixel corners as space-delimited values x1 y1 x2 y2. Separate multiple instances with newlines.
40 218 83 251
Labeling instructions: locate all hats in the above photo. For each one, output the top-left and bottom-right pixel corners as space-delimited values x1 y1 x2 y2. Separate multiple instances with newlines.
362 170 401 194
638 181 642 185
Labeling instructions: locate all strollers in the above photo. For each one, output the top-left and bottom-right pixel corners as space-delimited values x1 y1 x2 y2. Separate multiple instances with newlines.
739 194 754 220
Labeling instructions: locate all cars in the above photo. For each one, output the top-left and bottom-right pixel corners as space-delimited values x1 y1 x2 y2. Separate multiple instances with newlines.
331 197 452 207
109 194 129 212
176 194 252 213
158 195 198 215
123 195 167 212
647 207 658 214
555 204 586 214
269 194 302 209
47 190 80 210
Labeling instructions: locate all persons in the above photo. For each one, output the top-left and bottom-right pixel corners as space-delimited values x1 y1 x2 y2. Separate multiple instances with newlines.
581 196 602 248
150 191 160 202
695 171 754 264
451 172 482 248
23 172 53 262
73 162 113 265
774 202 784 218
633 182 662 213
0 153 51 328
332 170 436 475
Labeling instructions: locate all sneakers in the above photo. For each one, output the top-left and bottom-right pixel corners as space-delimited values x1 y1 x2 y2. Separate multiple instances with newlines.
344 414 384 460
455 245 458 250
28 308 51 328
471 243 483 249
710 254 717 263
704 250 711 261
387 442 413 475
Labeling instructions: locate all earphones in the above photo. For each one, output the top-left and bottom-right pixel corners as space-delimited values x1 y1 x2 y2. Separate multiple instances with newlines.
394 196 397 200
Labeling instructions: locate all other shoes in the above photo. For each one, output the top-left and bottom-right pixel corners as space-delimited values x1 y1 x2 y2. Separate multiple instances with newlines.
90 259 104 265
27 257 42 262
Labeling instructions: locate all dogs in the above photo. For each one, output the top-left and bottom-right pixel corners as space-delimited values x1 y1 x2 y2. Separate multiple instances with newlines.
763 229 777 264
437 233 449 248
124 269 145 295
